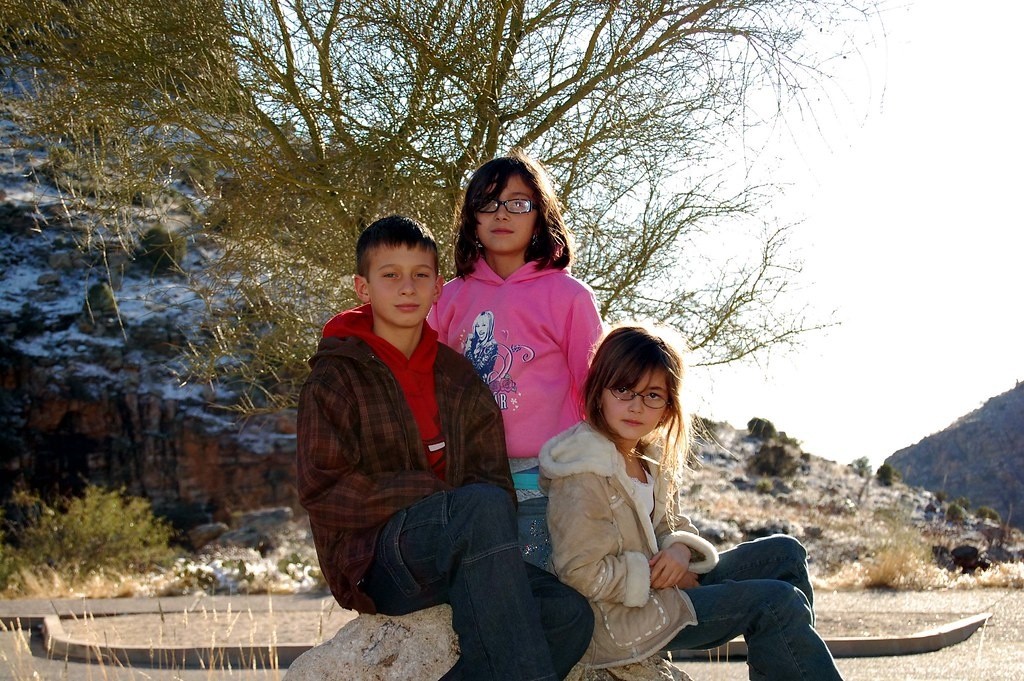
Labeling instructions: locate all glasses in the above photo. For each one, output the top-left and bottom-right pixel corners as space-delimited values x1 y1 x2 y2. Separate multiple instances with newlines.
604 387 671 409
474 198 540 213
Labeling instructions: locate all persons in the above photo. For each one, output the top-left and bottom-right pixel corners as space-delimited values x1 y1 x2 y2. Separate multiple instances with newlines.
424 155 603 573
294 216 595 680
536 325 843 681
